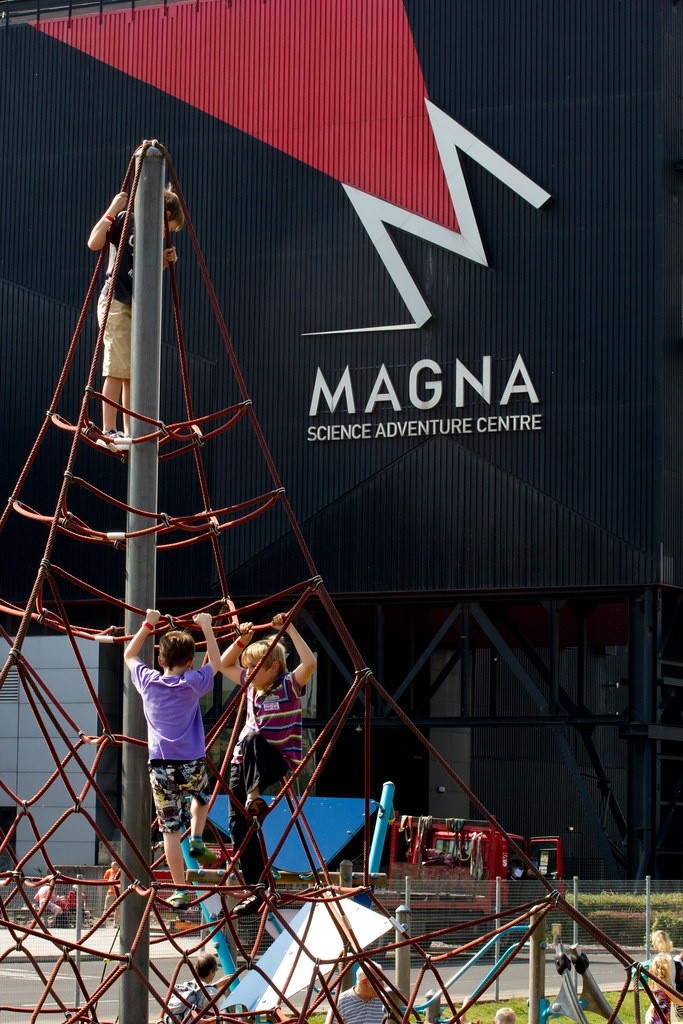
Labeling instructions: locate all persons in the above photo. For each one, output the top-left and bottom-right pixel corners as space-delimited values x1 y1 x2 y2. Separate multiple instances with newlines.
672 948 683 1024
63 884 87 928
164 953 235 1024
124 604 222 906
87 182 185 452
33 882 63 926
98 860 121 928
218 612 316 915
645 931 676 1024
325 960 385 1024
494 1007 516 1024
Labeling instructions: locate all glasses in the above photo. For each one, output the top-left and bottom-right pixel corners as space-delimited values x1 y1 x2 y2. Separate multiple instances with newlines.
213 967 219 971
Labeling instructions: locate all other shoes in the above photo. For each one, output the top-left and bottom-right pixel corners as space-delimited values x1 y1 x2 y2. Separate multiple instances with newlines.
244 797 267 829
233 896 278 917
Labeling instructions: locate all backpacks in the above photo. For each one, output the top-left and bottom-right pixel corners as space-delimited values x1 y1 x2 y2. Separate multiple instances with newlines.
165 984 213 1024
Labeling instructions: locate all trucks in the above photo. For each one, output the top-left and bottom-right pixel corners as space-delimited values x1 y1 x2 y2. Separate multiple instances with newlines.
150 816 565 953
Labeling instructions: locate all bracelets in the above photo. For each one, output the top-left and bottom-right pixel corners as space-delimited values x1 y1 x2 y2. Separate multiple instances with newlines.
141 620 156 631
236 638 245 649
101 212 115 224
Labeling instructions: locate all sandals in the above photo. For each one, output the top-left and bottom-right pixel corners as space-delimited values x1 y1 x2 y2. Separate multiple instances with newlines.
165 894 191 909
190 843 216 865
